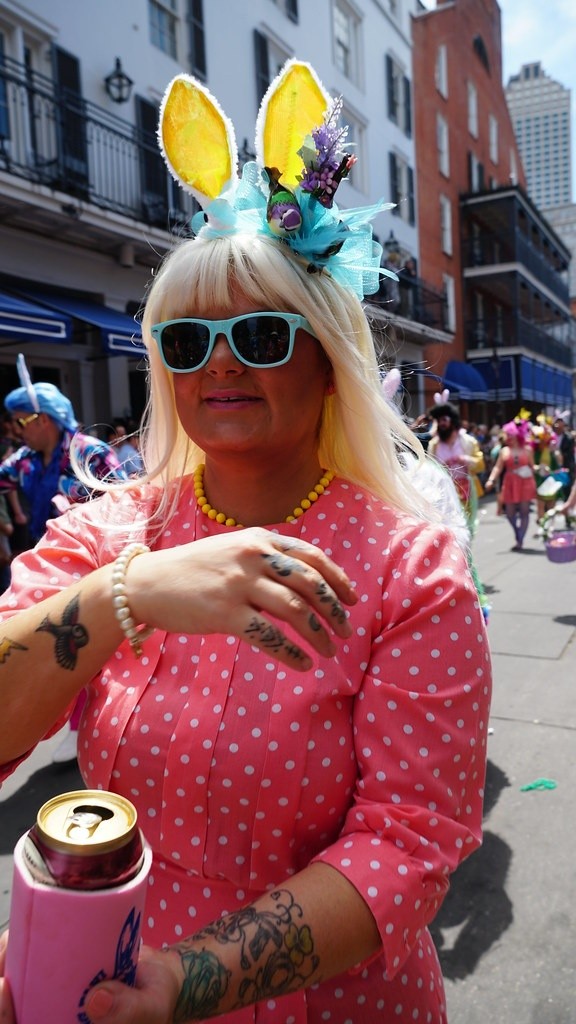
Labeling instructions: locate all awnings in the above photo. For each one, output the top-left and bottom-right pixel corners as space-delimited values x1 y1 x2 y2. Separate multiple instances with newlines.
443 361 489 401
400 361 468 395
0 293 75 349
23 291 146 362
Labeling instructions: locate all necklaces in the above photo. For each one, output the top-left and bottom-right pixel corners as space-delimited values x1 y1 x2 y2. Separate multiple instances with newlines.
194 464 336 528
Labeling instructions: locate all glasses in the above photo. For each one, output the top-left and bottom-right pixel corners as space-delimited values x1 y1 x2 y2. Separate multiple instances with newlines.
11 413 38 429
150 311 321 374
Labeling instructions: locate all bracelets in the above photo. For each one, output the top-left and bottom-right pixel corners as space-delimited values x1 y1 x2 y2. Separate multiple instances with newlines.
113 543 158 657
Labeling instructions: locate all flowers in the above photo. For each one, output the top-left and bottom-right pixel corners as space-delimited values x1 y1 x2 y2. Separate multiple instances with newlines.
295 95 358 212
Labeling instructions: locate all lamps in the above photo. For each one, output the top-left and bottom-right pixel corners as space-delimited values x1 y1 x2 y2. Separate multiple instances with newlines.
102 56 133 102
384 228 400 251
238 140 256 175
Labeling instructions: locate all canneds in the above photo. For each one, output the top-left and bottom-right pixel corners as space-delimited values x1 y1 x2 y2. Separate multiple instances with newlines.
19 789 144 890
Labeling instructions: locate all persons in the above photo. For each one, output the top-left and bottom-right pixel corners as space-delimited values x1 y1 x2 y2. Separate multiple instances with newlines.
483 407 575 550
0 223 492 1023
